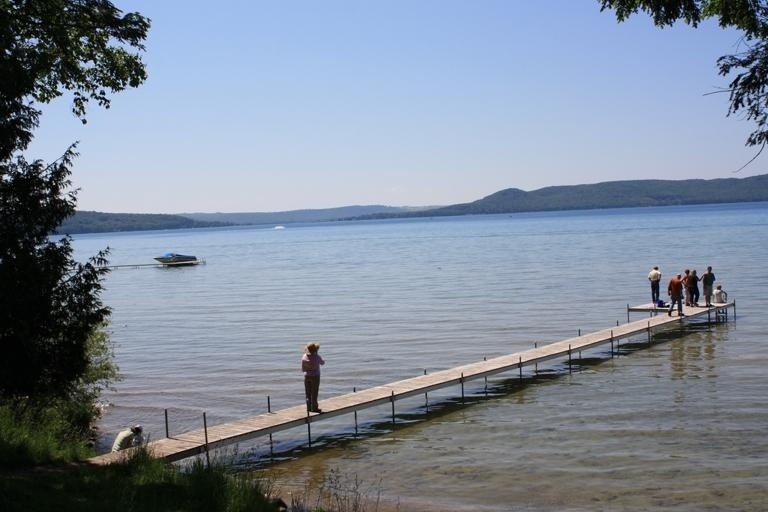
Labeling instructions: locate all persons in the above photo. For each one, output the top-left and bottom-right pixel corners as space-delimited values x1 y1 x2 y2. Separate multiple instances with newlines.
679 269 691 306
712 284 728 303
110 423 144 452
667 274 686 318
301 342 325 414
647 266 662 303
702 267 717 307
687 270 704 307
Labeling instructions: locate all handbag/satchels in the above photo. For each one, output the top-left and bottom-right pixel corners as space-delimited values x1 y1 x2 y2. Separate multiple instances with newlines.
657 300 664 307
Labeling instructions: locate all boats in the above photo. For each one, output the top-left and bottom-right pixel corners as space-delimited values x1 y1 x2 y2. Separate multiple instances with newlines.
154 253 197 265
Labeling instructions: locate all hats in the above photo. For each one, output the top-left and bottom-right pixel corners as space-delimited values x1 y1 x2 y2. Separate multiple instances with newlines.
303 342 320 355
